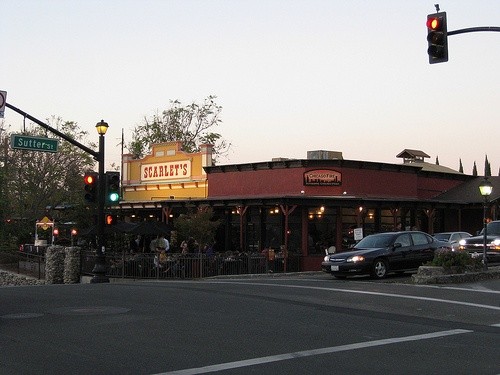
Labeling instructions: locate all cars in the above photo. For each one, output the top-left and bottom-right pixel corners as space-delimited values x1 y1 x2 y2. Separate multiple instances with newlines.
320 230 453 279
432 232 473 247
458 220 500 262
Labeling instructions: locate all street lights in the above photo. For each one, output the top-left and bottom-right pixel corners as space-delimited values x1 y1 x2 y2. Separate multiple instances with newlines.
478 176 494 267
90 119 110 284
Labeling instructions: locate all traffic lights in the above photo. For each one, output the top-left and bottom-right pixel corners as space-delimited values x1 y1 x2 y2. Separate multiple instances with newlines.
72 229 77 236
83 172 97 203
53 228 58 236
105 171 120 205
426 11 450 64
105 214 116 225
